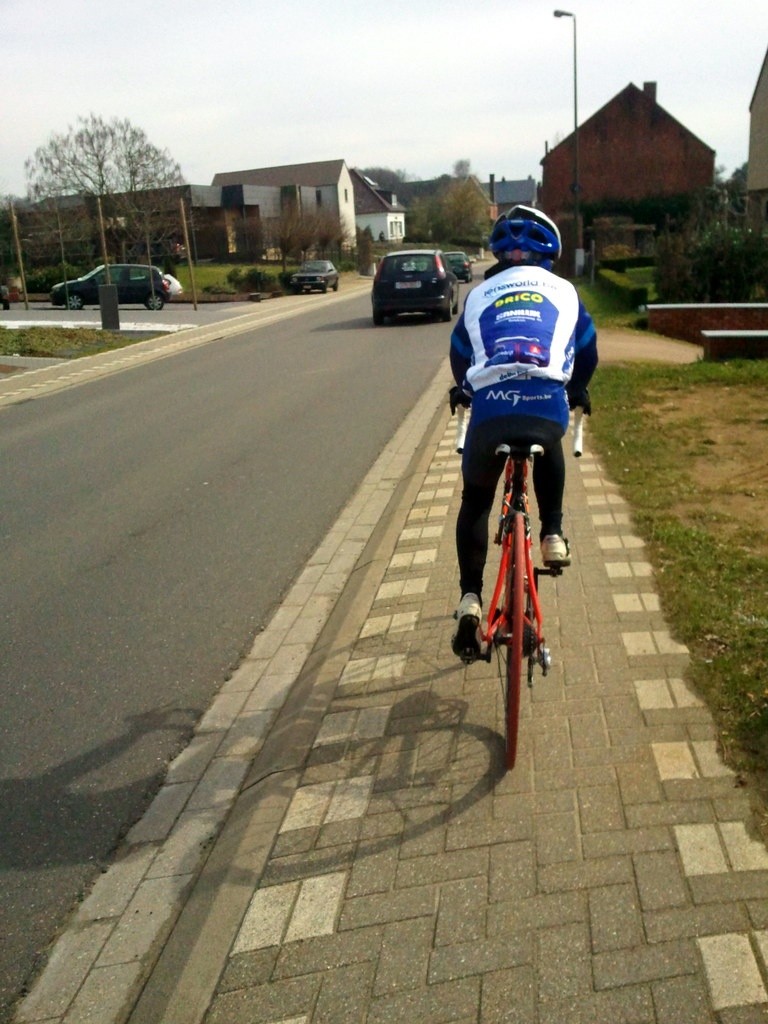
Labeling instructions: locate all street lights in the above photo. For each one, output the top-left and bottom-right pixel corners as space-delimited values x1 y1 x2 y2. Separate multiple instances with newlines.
551 8 579 272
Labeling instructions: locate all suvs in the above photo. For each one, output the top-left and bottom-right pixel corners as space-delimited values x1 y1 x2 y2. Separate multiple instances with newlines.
48 263 171 312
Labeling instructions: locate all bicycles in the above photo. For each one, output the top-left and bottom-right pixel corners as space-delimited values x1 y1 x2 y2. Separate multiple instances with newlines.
446 382 586 767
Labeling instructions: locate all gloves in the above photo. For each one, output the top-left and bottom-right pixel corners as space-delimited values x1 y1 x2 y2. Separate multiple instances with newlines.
450 388 472 416
568 387 591 416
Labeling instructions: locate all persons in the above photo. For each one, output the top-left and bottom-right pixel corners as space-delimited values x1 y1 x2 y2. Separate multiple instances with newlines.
448 205 599 657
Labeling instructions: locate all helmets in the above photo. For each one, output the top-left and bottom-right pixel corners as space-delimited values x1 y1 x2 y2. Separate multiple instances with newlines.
490 205 563 261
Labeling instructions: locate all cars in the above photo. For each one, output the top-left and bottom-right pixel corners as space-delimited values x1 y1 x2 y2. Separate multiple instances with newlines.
369 249 459 322
291 260 342 294
445 252 475 286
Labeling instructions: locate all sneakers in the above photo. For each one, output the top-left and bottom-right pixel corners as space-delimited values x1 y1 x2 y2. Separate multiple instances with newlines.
451 593 482 657
540 534 571 567
